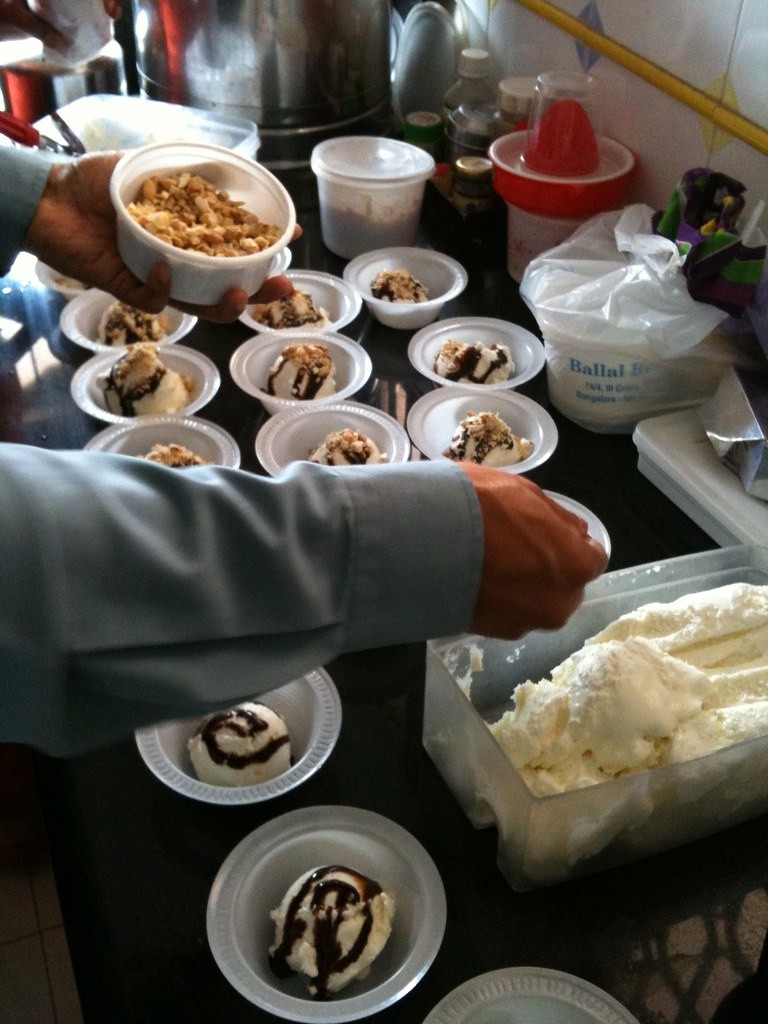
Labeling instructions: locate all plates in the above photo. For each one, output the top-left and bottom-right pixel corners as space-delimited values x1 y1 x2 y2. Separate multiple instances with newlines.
389 0 459 127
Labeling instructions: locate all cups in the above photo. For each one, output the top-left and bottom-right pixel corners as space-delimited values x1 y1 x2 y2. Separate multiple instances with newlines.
309 134 434 262
521 71 606 178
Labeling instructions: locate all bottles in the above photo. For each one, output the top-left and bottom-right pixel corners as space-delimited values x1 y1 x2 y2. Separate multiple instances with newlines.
407 44 543 223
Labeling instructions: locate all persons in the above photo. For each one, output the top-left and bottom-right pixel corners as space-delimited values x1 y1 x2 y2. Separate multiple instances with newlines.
0 0 122 64
0 143 609 761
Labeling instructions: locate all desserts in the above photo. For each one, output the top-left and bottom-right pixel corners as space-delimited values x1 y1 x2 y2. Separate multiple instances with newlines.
57 267 535 471
268 864 395 996
187 701 292 788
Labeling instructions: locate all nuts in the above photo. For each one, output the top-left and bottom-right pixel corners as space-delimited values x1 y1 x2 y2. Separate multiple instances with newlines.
124 169 280 259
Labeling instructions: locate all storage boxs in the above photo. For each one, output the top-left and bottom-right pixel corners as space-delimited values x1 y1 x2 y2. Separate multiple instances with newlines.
420 548 767 890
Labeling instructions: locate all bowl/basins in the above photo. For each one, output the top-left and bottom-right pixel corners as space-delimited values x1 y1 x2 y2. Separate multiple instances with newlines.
136 658 640 1024
61 141 612 574
